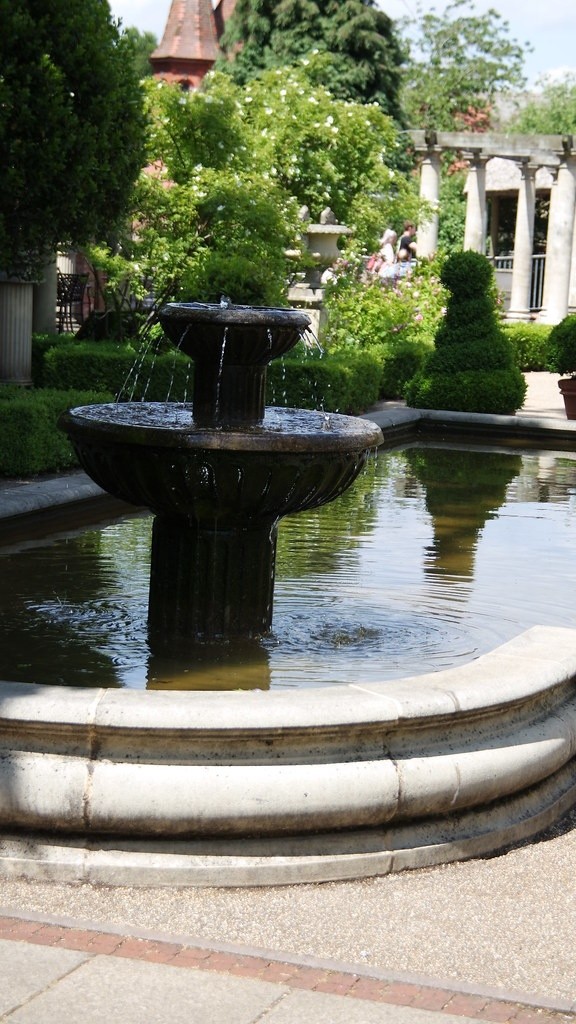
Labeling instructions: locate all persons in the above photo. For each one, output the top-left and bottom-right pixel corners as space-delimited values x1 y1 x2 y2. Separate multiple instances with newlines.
373 220 417 272
320 259 341 286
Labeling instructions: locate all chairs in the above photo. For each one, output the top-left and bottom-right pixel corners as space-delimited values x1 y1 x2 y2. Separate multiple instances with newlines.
54 272 89 334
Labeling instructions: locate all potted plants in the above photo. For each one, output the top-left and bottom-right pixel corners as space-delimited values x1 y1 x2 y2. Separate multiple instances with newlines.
543 313 576 421
